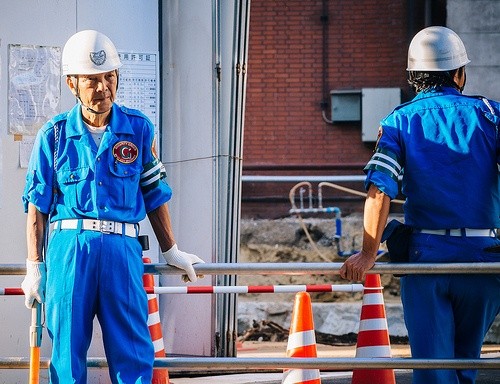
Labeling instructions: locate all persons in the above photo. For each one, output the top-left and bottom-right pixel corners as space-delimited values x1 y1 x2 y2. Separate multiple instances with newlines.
21 29 205 384
339 28 500 384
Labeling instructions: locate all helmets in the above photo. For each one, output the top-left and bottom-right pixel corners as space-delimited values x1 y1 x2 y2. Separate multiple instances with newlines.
61 30 125 76
406 25 471 72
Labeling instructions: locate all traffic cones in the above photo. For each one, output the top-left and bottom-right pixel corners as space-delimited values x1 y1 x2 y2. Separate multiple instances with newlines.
142 258 170 384
281 292 321 384
352 274 395 384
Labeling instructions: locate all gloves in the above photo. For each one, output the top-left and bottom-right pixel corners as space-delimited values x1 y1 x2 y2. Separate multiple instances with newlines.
21 259 45 309
161 243 205 283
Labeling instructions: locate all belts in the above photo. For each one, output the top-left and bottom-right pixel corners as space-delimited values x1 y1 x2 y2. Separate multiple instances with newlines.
50 218 138 238
421 228 498 238
